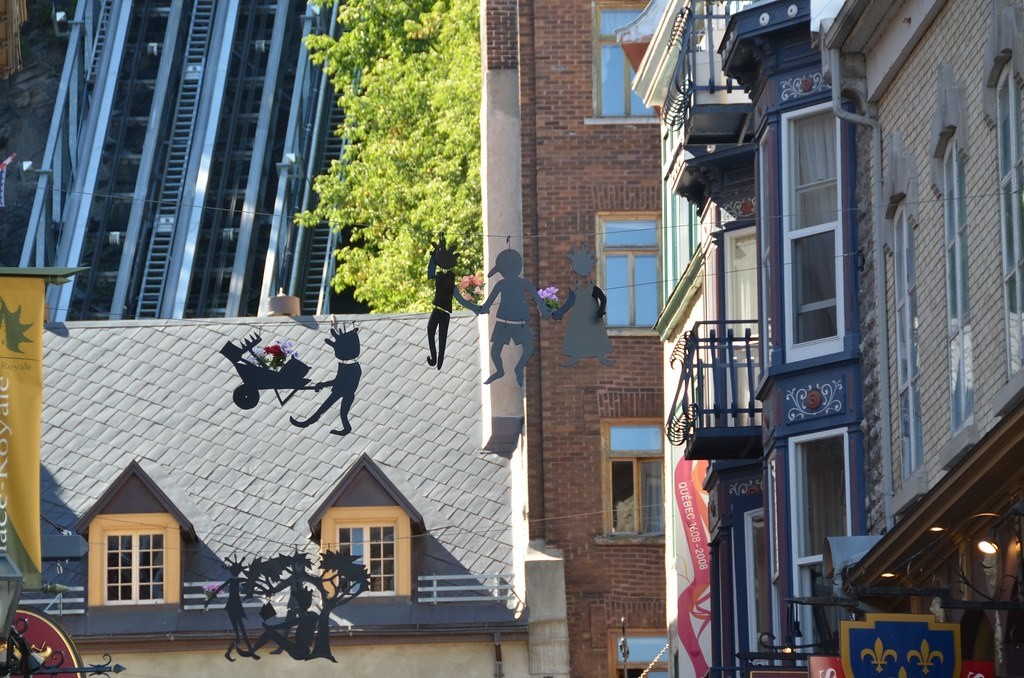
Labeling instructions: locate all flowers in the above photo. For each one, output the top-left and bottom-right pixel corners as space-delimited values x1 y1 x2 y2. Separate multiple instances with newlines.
458 271 487 306
248 341 299 373
201 581 224 614
534 287 562 319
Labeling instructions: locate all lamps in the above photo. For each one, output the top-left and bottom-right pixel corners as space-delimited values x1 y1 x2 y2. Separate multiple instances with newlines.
977 523 1001 556
787 604 804 639
